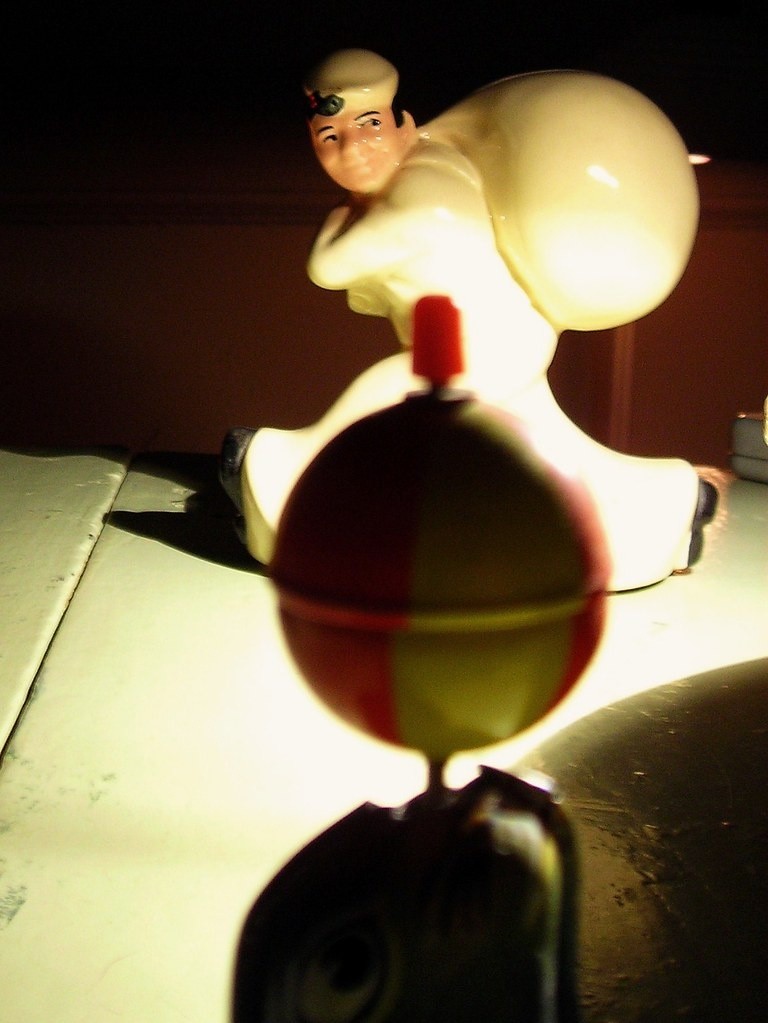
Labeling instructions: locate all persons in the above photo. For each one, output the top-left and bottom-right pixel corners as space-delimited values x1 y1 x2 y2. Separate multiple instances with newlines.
218 48 720 592
